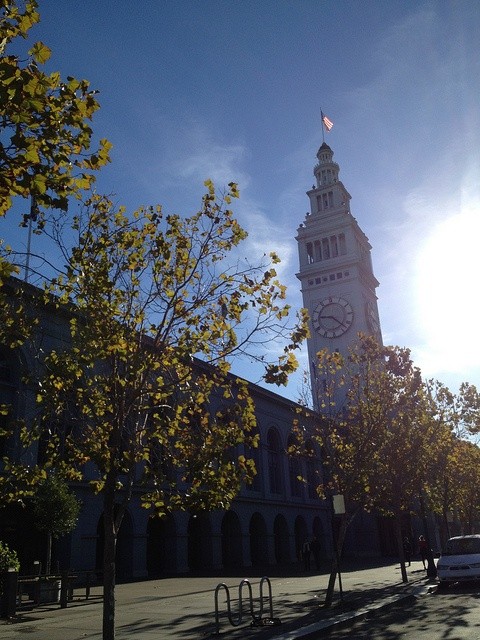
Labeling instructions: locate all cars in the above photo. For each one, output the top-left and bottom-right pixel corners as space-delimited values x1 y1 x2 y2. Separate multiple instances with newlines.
437 534 480 588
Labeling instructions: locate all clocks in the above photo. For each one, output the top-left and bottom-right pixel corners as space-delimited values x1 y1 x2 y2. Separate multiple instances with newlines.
311 296 353 338
367 301 379 334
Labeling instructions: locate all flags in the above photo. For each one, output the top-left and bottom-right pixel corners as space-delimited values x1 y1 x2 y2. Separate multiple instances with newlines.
321 110 335 133
